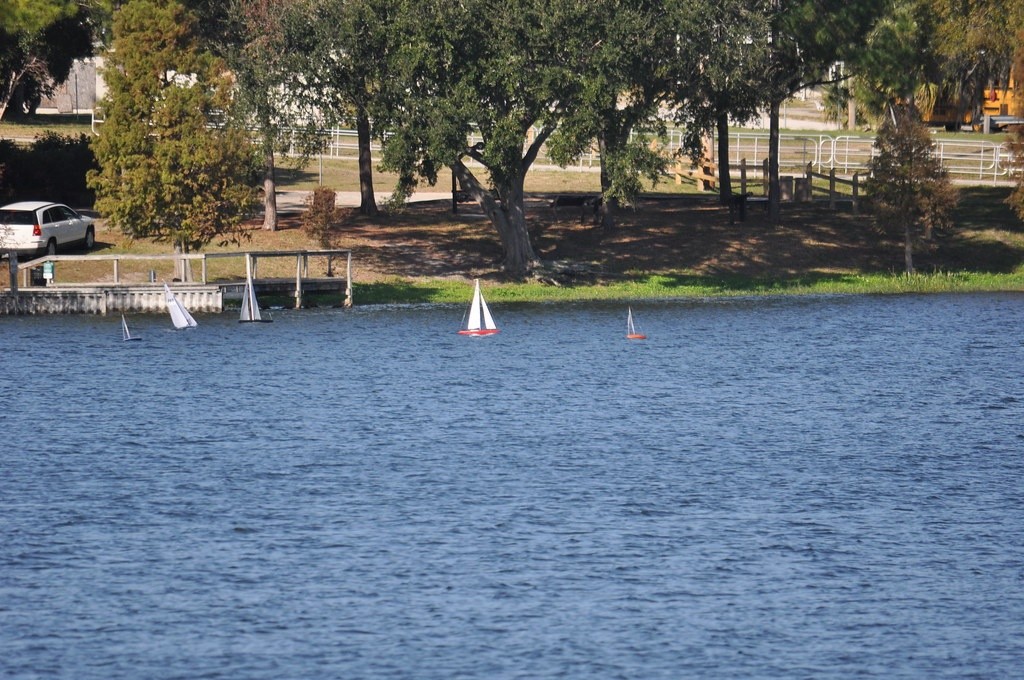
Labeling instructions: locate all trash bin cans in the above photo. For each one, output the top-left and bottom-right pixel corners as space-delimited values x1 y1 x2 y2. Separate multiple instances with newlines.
30 266 46 286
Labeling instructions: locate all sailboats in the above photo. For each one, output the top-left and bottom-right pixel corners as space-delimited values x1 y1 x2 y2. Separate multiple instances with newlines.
120 313 143 341
628 307 646 340
238 253 274 323
455 279 500 336
164 283 198 331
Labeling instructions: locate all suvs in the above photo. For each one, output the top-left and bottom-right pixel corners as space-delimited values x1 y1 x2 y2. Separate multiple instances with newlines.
0 203 96 261
